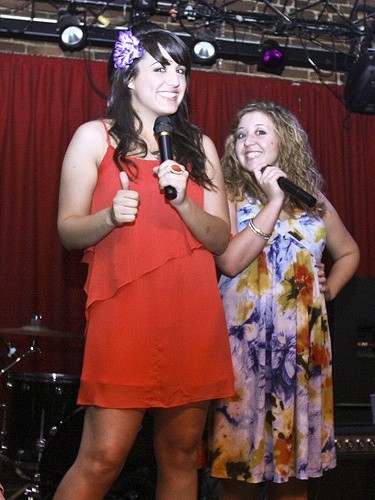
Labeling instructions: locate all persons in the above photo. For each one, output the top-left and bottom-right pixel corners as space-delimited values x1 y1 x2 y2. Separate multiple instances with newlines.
54 25 230 500
214 99 357 500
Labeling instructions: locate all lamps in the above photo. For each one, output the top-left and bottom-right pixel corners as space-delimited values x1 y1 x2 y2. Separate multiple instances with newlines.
257 23 289 76
56 0 89 52
189 6 221 66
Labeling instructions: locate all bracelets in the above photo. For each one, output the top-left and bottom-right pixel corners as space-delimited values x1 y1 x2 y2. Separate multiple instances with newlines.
249 219 272 240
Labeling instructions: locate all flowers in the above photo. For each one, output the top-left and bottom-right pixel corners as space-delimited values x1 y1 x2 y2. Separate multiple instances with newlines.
113 29 144 72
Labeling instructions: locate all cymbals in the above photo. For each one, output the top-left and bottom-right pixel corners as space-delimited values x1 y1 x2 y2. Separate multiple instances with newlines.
0 325 84 339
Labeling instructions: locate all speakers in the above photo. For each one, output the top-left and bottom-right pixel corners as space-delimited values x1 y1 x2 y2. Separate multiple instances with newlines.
326 275 375 408
306 425 375 500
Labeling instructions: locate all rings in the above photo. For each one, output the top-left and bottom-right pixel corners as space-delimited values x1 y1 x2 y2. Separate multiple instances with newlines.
169 165 183 174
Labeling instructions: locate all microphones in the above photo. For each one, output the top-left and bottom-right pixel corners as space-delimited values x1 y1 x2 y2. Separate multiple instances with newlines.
261 165 316 207
153 116 178 200
0 336 20 360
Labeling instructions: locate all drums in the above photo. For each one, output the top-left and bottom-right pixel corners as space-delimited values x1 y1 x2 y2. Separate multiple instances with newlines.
38 403 204 500
0 370 86 471
